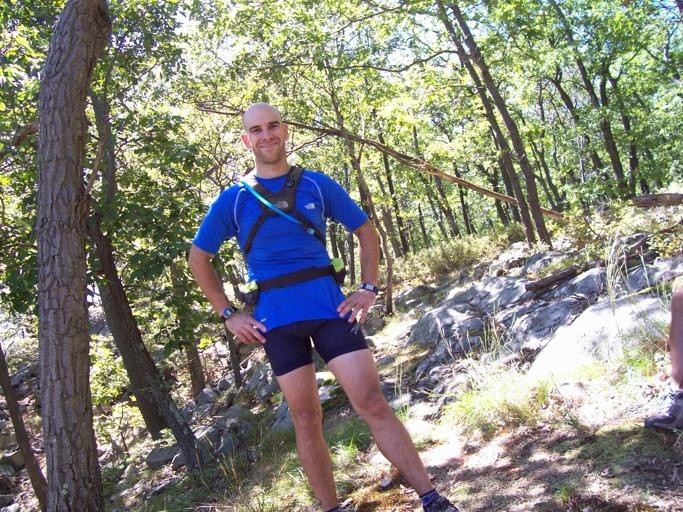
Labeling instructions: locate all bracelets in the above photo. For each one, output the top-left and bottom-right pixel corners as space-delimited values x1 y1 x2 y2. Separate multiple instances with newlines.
360 283 379 296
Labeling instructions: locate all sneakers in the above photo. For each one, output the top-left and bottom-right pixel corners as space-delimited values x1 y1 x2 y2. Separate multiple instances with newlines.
422 494 459 512
644 389 683 429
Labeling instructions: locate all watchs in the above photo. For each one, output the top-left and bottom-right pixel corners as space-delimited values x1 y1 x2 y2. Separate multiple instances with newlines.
220 306 238 322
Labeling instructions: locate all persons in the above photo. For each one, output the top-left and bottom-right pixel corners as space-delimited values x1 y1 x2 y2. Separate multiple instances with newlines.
643 286 683 430
187 102 463 512
